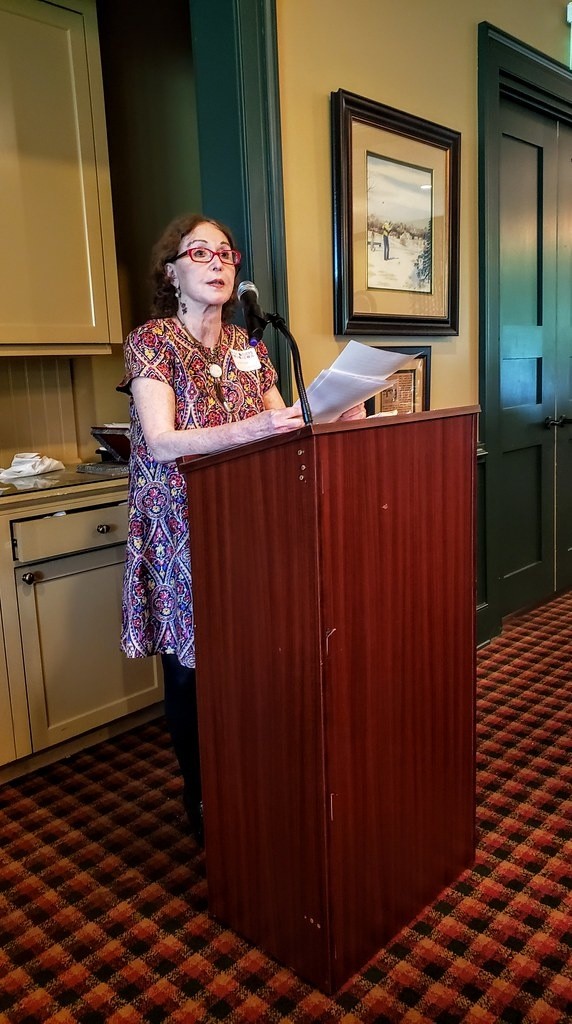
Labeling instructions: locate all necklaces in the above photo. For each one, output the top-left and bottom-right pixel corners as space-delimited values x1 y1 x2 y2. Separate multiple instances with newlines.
176 314 231 412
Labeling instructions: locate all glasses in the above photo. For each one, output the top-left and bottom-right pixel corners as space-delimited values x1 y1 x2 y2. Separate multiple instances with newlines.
168 247 242 265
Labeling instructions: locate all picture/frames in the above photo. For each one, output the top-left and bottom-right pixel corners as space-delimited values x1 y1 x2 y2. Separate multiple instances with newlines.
331 88 459 336
364 346 432 418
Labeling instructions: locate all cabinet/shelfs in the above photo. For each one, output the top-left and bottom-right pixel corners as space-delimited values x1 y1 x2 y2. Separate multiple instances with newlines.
0 0 124 356
0 502 166 768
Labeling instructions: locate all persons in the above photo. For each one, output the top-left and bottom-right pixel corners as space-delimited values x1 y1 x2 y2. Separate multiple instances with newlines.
382 220 393 260
116 214 366 843
396 222 404 239
371 230 375 251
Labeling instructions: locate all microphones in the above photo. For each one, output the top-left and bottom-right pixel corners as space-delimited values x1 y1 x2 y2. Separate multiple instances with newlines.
238 281 261 347
